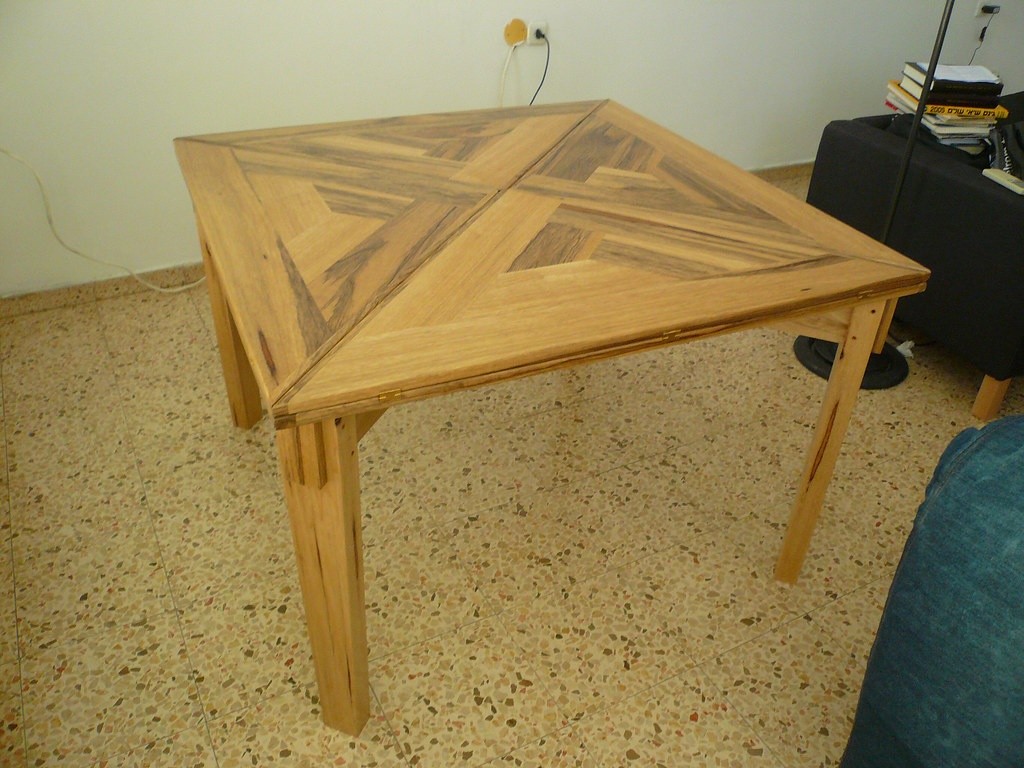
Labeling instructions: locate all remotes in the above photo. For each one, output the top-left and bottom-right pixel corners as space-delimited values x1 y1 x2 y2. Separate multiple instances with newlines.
983 169 1024 195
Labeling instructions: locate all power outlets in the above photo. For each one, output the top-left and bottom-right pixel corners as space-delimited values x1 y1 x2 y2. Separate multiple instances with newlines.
975 0 992 17
527 22 548 46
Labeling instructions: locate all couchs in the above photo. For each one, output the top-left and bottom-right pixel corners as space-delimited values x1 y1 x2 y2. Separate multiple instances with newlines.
805 91 1024 420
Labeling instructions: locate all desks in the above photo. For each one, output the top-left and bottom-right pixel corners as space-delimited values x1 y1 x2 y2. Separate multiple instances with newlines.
172 99 932 740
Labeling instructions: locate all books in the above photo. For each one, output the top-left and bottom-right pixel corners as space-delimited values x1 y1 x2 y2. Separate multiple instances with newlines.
883 60 1011 145
